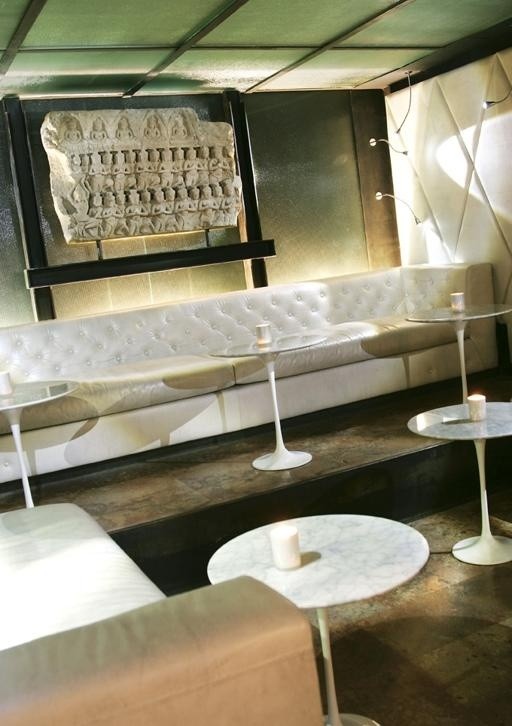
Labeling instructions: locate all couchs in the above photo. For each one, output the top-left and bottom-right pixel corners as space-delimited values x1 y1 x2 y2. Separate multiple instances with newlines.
1 501 328 726
0 259 496 489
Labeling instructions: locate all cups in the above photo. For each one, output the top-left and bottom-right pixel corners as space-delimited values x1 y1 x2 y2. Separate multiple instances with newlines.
256 324 273 345
450 292 465 313
0 371 15 396
467 393 486 421
270 523 303 570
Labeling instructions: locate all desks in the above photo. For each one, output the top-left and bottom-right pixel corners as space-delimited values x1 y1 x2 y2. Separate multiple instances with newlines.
1 380 80 508
206 512 431 725
210 333 326 471
405 401 512 566
405 302 512 403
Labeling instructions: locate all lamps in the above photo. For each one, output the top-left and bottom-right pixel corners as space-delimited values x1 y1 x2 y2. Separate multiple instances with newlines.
370 138 409 157
483 85 512 110
376 191 422 227
394 71 415 134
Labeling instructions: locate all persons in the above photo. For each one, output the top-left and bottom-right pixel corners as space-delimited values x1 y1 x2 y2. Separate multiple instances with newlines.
64 115 239 239
